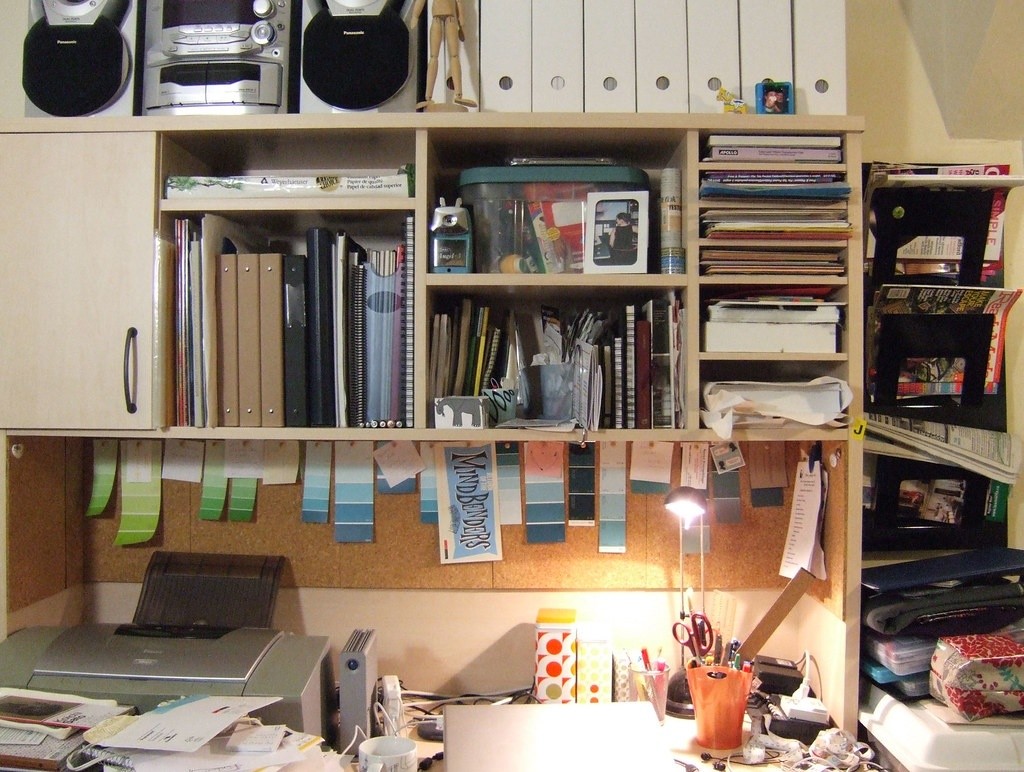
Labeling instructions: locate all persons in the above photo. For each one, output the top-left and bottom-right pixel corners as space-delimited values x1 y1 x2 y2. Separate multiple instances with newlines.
763 86 784 112
410 0 477 109
609 212 634 249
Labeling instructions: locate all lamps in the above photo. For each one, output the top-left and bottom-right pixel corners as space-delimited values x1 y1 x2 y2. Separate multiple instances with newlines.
665 487 706 718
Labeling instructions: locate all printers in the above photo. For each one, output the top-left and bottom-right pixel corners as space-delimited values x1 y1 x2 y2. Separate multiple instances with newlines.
0 549 335 750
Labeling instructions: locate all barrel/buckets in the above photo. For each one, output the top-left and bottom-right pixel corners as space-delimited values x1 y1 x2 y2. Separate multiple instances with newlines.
686 656 753 751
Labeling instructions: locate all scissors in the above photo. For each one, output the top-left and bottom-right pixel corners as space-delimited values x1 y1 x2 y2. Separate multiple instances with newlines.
672 614 713 661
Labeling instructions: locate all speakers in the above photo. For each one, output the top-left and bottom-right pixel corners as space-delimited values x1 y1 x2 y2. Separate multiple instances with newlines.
300 0 426 113
21 0 139 117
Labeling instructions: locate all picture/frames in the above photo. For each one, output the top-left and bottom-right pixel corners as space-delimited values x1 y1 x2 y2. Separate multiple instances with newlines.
584 190 649 274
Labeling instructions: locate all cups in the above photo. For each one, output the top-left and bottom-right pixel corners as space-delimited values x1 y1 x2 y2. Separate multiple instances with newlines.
628 660 670 726
358 736 416 772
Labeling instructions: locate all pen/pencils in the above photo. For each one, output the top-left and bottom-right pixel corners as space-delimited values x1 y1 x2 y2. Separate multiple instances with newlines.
714 636 755 670
641 648 653 671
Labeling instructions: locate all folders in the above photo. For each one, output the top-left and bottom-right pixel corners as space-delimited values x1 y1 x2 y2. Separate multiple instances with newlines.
217 254 305 428
308 228 338 427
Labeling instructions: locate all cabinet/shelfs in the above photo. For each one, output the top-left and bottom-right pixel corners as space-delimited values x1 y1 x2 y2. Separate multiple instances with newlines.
0 113 867 772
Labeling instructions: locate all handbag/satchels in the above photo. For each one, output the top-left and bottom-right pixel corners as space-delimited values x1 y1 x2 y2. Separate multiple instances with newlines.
930 632 1023 722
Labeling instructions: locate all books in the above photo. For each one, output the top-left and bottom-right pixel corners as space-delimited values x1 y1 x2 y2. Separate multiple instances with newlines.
602 288 686 429
862 159 1023 593
151 212 415 429
0 695 326 772
704 295 848 353
699 133 853 275
429 298 507 428
